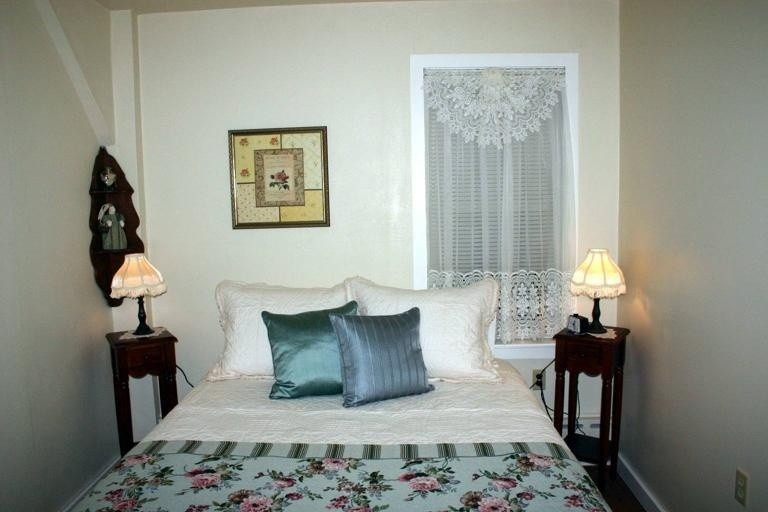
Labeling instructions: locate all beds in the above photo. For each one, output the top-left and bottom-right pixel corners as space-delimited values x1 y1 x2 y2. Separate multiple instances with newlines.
60 358 614 512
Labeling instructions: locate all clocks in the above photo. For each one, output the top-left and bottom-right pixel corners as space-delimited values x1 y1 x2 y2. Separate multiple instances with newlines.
568 314 588 333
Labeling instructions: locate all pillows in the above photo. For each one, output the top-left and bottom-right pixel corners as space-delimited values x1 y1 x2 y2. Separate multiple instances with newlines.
205 277 347 383
262 300 359 400
328 307 437 408
344 278 502 386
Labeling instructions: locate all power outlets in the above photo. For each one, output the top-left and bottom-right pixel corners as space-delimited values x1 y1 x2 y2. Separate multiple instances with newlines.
533 369 545 391
734 468 749 506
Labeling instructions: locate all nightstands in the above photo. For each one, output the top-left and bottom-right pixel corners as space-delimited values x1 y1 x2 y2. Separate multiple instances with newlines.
106 327 178 460
553 325 631 485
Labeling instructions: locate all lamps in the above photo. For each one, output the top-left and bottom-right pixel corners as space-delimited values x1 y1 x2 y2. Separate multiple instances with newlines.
570 249 626 333
107 251 167 336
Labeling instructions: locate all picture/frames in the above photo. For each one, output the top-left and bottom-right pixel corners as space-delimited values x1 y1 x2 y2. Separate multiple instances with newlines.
229 126 330 229
